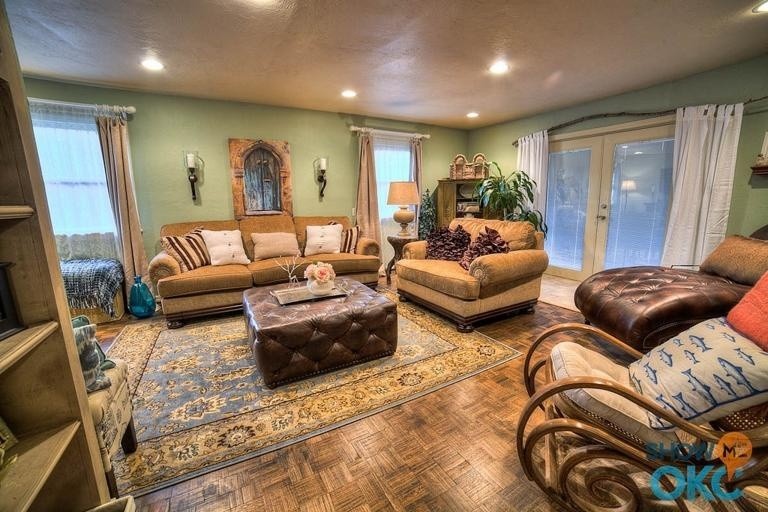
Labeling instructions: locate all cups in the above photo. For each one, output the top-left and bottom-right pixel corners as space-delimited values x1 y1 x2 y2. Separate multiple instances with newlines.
457 202 464 211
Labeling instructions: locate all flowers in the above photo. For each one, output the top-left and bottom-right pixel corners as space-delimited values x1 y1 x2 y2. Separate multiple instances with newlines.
303 261 336 285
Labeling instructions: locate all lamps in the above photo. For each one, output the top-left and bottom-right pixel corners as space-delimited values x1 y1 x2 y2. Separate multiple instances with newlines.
386 181 422 237
182 150 204 200
313 156 328 197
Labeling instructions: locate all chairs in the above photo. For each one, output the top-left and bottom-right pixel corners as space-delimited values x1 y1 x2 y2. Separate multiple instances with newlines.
515 268 768 512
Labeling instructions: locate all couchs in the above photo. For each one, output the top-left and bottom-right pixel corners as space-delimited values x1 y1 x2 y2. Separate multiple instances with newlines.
86 357 138 500
148 215 381 329
395 218 549 332
573 225 768 351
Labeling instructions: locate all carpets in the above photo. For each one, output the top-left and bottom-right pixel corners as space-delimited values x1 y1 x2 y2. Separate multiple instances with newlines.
103 283 523 500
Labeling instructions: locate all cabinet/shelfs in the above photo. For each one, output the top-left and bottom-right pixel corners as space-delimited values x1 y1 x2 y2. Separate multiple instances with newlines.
0 1 112 512
436 178 504 228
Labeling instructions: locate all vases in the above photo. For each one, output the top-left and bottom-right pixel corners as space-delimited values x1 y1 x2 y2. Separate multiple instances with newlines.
129 275 156 319
307 281 335 295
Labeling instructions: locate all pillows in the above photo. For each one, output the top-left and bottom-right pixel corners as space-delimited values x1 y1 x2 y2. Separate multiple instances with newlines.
725 266 768 349
327 221 360 255
628 315 768 434
202 229 250 266
251 232 301 261
425 224 471 261
700 235 767 287
304 224 344 256
161 224 211 273
75 324 111 394
458 226 511 272
70 314 114 370
